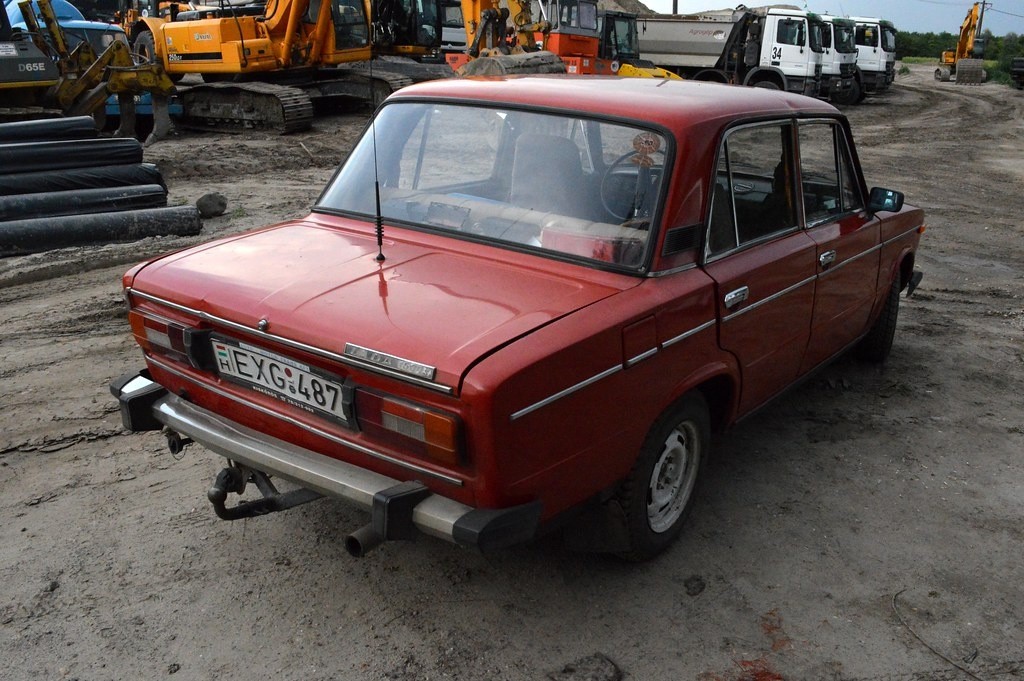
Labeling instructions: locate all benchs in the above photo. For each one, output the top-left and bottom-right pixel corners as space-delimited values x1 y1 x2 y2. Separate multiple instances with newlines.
372 184 649 260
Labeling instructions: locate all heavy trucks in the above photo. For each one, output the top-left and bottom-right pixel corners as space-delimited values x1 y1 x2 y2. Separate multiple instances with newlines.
632 4 900 105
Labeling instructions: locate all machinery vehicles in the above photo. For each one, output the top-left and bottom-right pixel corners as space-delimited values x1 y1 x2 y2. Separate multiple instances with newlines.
0 0 689 137
932 1 989 87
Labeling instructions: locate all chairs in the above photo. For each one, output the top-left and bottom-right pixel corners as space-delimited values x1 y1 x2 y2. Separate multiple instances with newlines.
640 173 736 254
511 134 589 217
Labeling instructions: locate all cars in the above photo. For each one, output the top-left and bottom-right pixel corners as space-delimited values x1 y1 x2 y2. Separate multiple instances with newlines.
110 73 930 563
